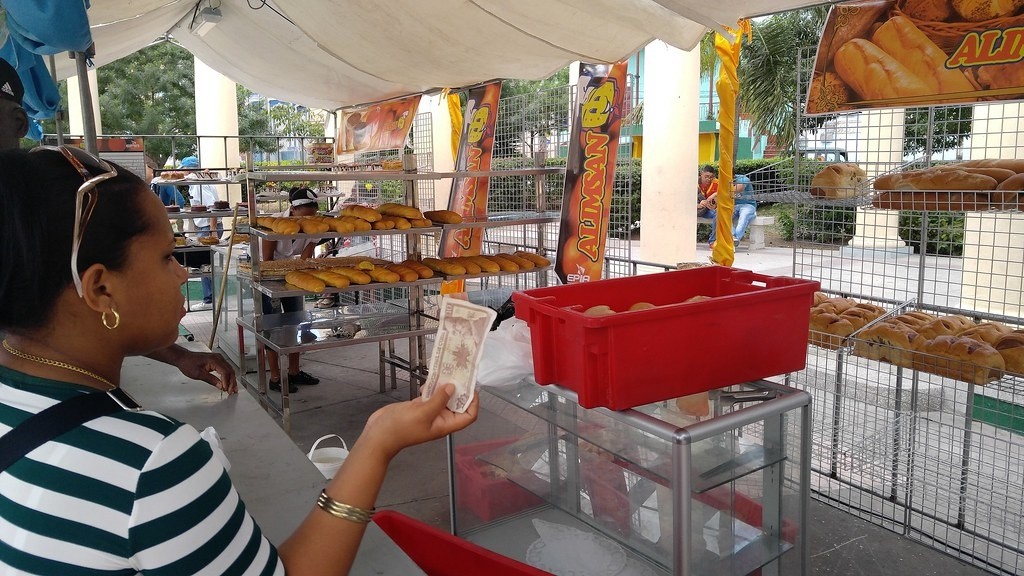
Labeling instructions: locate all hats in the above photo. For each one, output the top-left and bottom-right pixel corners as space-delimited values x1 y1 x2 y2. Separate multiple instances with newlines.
177 156 199 170
0 58 25 106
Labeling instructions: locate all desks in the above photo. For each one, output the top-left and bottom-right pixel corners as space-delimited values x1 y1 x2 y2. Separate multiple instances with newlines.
119 342 428 576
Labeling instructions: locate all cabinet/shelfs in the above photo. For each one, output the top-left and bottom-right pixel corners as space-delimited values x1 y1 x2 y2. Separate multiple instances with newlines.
172 246 213 313
237 165 565 430
152 178 248 252
446 375 813 576
790 43 1024 576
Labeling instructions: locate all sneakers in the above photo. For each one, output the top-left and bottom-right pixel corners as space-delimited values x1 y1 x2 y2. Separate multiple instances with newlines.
190 302 213 311
269 378 298 393
287 371 319 385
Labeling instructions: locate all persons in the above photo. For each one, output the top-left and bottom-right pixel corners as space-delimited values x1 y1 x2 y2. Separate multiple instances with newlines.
247 187 322 392
697 165 757 250
159 184 185 232
314 198 363 308
0 143 480 576
0 57 29 152
176 157 223 310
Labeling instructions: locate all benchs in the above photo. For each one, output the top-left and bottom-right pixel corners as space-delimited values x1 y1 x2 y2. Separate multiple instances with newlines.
696 217 775 249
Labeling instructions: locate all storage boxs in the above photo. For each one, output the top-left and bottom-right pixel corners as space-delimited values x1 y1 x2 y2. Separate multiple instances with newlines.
453 437 546 523
511 265 820 411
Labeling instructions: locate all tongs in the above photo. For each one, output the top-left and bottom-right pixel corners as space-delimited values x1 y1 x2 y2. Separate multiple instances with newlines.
708 390 775 406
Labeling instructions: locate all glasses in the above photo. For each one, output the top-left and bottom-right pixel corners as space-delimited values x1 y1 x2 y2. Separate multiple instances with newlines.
30 143 118 301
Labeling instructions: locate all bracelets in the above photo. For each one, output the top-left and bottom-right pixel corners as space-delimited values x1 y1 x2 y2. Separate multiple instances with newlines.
317 488 376 524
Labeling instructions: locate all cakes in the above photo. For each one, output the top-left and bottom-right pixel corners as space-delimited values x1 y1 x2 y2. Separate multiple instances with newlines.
174 237 187 246
165 205 180 212
232 234 251 244
190 204 207 212
161 170 185 179
213 200 229 209
198 236 220 245
238 202 248 210
193 168 219 179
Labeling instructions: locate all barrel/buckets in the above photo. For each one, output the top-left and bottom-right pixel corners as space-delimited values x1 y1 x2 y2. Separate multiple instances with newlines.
305 143 335 163
306 434 349 479
401 153 417 174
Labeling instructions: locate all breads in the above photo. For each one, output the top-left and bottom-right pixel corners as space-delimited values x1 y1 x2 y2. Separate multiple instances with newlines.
805 0 1024 117
259 203 464 236
872 158 1024 213
805 291 1023 387
238 250 552 290
585 295 714 316
809 162 870 199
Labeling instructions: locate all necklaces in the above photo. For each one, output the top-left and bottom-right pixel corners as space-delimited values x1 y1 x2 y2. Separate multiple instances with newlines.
3 339 117 388
